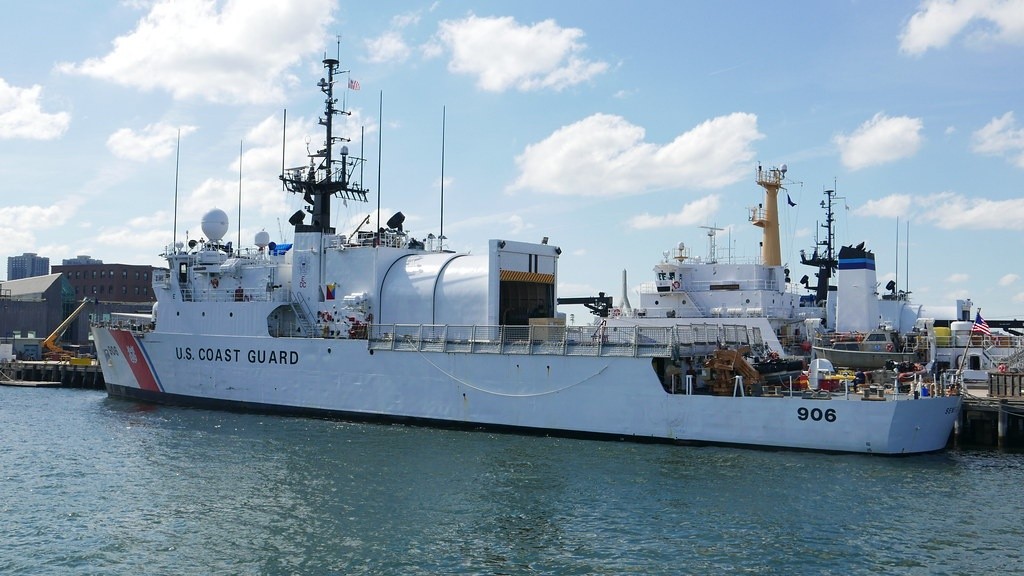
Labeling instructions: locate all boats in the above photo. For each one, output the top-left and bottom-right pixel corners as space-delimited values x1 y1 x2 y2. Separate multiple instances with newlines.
90 35 966 456
812 329 916 369
557 160 826 344
786 175 1024 381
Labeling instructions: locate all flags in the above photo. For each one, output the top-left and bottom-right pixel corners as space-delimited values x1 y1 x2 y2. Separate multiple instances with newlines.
348 79 360 90
972 313 990 336
788 195 797 207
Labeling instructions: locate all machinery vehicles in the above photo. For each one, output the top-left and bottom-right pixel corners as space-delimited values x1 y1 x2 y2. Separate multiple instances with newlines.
42 296 98 361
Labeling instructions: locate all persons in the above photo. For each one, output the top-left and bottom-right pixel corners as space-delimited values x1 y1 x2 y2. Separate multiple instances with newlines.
851 368 865 393
